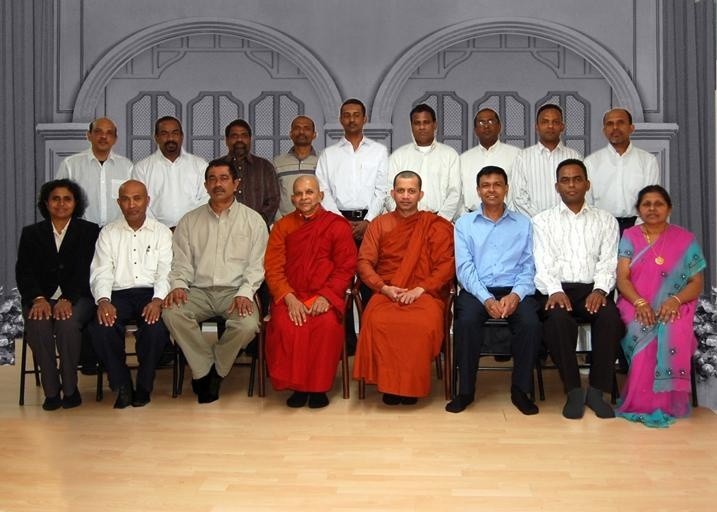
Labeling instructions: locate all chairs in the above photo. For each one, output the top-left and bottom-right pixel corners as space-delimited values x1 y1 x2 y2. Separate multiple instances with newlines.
255 286 351 399
351 275 457 401
456 293 535 402
535 287 619 408
99 301 177 404
20 293 101 410
179 307 256 401
618 313 699 411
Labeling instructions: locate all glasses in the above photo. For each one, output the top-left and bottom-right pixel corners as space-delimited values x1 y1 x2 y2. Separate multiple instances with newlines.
477 119 496 128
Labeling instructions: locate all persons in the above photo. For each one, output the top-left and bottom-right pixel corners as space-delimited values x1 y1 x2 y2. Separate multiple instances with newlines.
14 99 706 419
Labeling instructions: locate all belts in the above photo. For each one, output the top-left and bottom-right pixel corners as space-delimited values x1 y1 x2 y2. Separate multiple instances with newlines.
340 210 367 218
